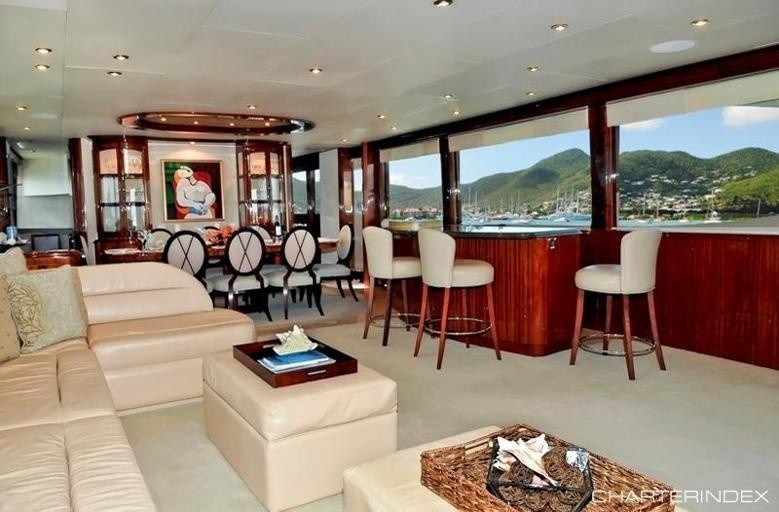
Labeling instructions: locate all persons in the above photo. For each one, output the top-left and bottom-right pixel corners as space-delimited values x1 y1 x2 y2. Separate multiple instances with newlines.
173 164 216 219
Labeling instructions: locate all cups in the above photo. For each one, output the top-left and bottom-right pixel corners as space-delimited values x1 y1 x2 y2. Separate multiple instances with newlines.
137 229 154 252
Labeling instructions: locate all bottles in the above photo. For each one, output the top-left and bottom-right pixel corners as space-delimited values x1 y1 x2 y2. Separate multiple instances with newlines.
275 215 282 237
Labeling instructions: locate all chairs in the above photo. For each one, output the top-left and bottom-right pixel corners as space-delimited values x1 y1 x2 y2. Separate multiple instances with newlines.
262 226 325 319
312 224 359 303
413 228 501 370
362 226 436 346
568 228 666 380
389 219 416 230
418 219 442 228
74 233 91 265
201 226 224 281
210 227 273 321
163 230 215 307
143 228 173 253
250 226 287 276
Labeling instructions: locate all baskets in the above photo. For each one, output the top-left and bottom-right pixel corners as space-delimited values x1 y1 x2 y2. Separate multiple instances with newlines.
421 422 676 512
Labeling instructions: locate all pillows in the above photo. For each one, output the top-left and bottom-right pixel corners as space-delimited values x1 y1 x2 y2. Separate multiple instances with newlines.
3 264 88 354
0 277 21 363
0 247 28 276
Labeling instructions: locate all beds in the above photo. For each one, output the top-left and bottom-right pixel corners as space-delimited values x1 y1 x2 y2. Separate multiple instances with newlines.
25 249 80 270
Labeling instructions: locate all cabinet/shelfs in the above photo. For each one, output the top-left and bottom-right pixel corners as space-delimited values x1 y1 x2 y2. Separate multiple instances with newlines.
92 134 152 240
235 140 293 232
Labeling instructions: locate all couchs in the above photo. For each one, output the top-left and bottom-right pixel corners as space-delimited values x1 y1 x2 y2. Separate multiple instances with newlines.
203 347 397 511
0 268 158 512
80 262 257 420
343 423 688 512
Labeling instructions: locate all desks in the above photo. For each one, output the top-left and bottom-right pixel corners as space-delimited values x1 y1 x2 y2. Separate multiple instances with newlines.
386 224 584 358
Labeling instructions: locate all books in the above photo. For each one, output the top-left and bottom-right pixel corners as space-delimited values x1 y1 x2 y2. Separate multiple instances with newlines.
258 351 336 377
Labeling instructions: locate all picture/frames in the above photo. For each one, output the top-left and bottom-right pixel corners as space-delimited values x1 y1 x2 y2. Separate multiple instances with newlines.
161 158 225 223
